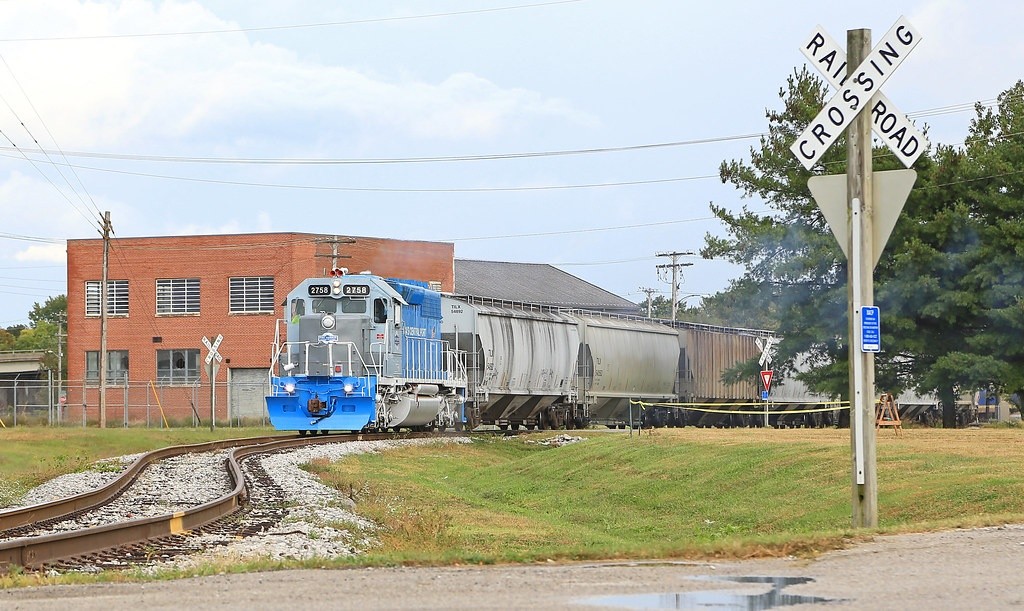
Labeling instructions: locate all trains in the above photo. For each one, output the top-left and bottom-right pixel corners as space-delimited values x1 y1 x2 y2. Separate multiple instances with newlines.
260 268 1023 434
